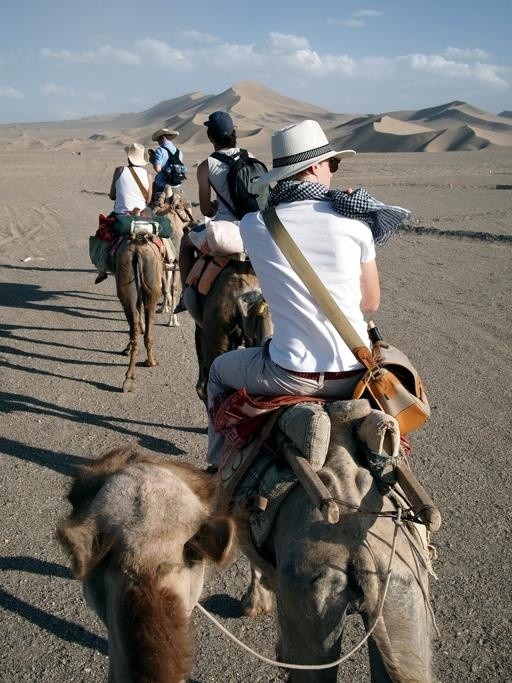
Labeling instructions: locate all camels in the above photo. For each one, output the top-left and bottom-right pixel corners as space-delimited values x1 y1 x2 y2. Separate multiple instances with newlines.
48 184 442 683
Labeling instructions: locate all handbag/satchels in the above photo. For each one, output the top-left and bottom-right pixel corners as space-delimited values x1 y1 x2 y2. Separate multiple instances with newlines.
351 338 430 437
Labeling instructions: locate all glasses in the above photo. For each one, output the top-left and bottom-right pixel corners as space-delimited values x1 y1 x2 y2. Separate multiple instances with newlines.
327 157 342 173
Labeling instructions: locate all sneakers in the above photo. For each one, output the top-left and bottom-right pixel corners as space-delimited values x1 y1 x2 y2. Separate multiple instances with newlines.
172 295 187 315
94 271 107 285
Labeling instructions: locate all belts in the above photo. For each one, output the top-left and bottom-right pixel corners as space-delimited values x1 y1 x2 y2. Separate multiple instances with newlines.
276 366 363 381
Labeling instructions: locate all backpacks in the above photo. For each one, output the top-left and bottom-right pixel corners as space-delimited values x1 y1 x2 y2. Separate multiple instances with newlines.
160 144 187 187
207 149 271 219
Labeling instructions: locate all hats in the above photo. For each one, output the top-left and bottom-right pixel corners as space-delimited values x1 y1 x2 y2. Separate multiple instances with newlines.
256 119 358 186
124 142 151 167
152 128 179 142
203 111 235 137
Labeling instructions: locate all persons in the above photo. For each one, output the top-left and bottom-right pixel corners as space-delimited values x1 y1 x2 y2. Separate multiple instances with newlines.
92 142 178 286
205 115 413 467
146 127 185 209
172 110 256 314
